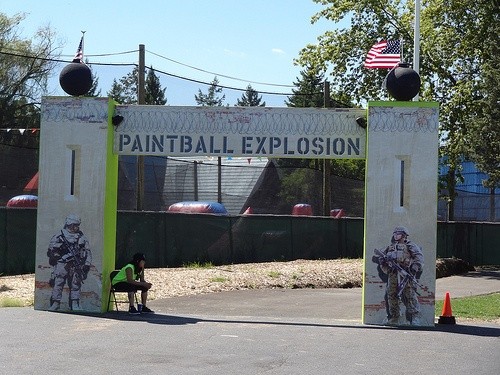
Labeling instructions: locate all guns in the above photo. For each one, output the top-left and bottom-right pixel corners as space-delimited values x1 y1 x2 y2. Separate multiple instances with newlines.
56 234 87 279
374 248 431 297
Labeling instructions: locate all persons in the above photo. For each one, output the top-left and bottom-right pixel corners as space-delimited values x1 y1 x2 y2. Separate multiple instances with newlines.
47 215 92 311
374 224 424 325
110 252 155 315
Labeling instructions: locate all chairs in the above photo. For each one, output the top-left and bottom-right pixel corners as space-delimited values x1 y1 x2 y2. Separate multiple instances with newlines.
107 271 140 314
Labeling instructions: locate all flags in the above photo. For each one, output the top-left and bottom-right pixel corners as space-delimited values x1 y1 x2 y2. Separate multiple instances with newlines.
362 38 404 68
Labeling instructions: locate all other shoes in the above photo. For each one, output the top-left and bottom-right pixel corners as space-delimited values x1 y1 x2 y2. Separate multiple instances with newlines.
129 306 140 315
141 305 153 313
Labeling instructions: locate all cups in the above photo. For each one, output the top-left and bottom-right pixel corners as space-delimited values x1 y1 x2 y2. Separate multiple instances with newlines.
138 304 143 312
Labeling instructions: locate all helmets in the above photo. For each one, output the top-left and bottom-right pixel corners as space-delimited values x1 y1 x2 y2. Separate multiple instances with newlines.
65 216 82 225
393 226 409 236
